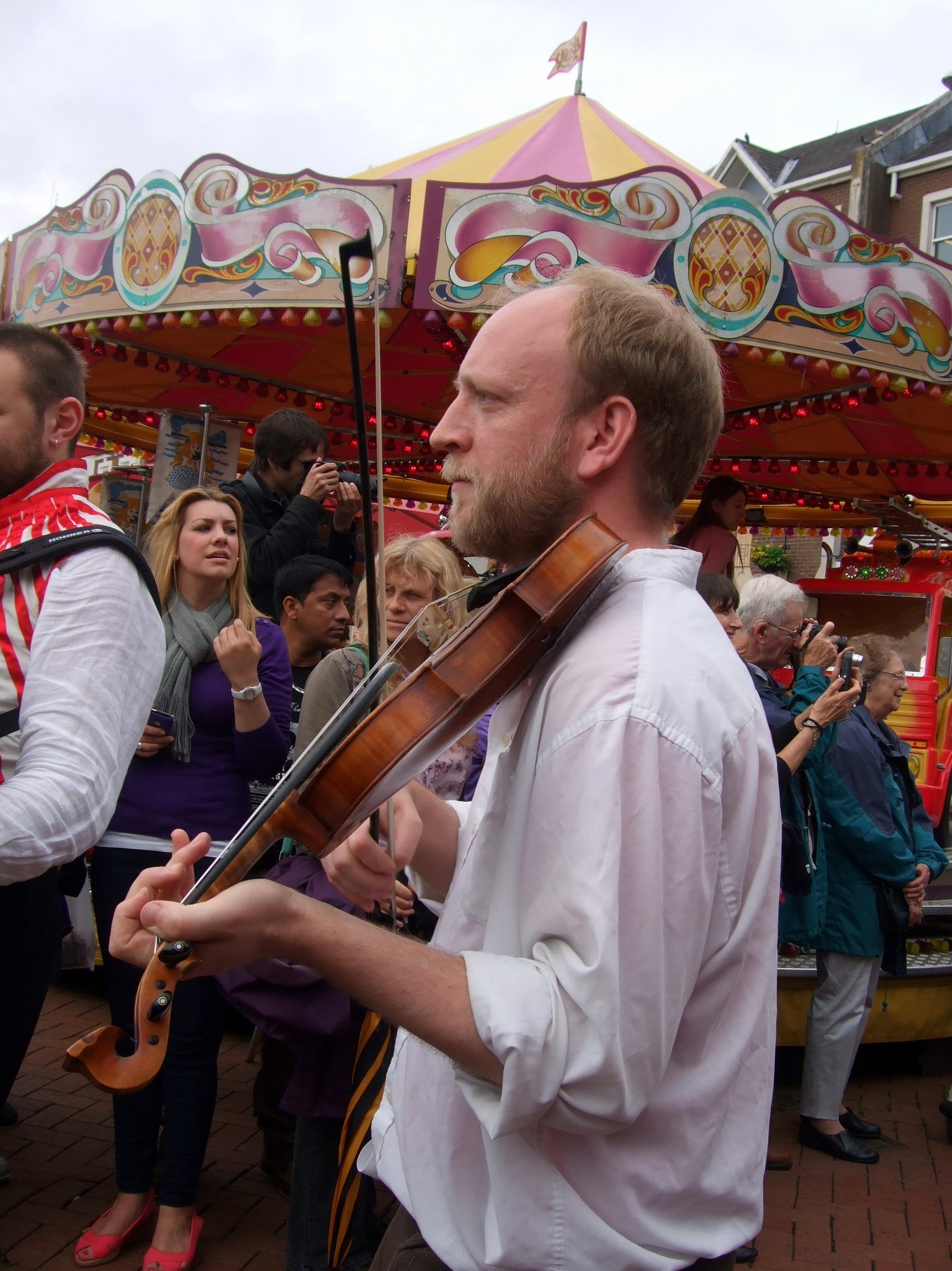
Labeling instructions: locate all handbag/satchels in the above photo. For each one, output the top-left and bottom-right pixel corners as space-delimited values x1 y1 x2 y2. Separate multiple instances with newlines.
874 878 909 938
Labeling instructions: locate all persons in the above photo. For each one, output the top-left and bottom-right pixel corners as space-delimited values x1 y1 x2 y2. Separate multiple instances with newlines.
110 265 782 1271
670 475 949 1169
0 322 499 1270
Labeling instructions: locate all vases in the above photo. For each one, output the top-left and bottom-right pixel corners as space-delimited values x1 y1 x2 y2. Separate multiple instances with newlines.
758 563 779 573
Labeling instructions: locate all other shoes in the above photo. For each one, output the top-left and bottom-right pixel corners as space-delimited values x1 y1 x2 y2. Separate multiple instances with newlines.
840 1106 882 1139
797 1117 880 1163
764 1143 792 1171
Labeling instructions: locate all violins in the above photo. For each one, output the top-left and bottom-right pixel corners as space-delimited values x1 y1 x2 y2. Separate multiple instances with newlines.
60 509 632 1098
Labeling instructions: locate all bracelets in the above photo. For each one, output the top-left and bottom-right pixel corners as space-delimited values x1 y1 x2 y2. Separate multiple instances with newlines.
801 717 824 736
799 719 819 750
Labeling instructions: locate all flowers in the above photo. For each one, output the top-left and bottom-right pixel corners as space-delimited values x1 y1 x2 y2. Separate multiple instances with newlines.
749 545 793 572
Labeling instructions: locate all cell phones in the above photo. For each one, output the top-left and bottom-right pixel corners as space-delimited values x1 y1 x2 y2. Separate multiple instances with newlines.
147 708 175 736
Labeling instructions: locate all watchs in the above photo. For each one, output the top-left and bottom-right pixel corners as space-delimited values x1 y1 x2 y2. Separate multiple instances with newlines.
231 682 263 700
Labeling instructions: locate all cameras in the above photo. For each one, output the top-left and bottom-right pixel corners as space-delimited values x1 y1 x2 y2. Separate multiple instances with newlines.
838 652 864 695
306 460 388 502
803 624 848 651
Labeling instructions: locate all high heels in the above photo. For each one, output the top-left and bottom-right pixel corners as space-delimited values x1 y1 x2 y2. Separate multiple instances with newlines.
143 1211 204 1271
75 1200 157 1265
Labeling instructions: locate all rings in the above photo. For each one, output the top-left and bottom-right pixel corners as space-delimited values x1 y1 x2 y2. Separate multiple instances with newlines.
852 700 857 708
135 741 143 750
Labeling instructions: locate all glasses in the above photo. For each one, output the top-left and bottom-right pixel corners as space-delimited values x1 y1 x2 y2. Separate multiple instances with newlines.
878 670 907 681
297 455 314 472
747 622 800 640
